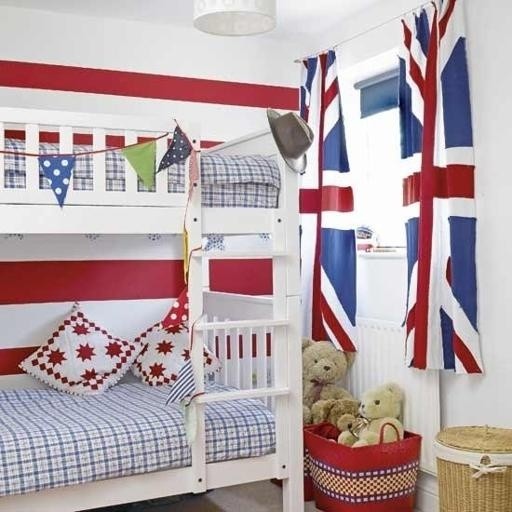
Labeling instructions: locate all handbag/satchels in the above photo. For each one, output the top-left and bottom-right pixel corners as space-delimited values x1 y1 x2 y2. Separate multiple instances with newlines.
269 420 422 511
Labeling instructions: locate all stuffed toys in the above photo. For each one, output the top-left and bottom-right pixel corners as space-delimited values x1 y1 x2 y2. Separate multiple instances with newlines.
301 335 404 447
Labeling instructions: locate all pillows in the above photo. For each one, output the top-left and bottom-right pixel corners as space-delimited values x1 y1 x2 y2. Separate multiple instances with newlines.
17 297 225 396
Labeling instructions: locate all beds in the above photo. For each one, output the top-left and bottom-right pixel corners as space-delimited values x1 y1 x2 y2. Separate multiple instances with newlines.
0 106 306 511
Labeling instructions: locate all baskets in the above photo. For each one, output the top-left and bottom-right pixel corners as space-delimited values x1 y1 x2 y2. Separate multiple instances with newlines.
432 422 512 511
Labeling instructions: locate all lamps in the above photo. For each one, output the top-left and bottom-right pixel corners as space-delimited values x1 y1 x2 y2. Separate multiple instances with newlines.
191 1 277 38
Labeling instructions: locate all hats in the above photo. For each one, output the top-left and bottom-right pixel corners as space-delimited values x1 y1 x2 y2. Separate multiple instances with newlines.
265 105 314 175
